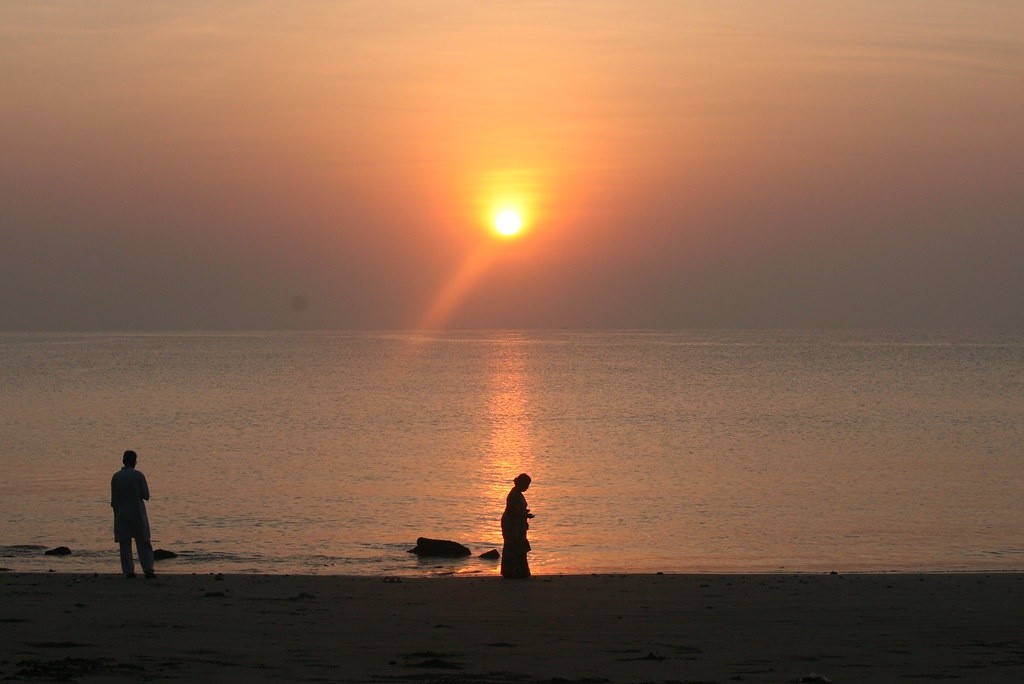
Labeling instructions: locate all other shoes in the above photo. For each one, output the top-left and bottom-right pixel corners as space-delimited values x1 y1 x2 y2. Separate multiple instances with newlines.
127 572 136 578
145 572 157 579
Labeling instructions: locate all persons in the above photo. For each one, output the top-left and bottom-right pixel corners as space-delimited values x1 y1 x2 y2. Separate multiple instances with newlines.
110 448 158 581
500 473 536 580
